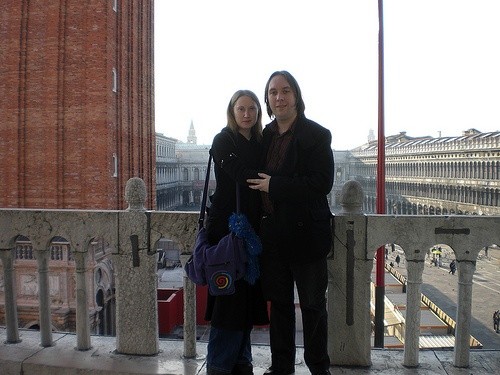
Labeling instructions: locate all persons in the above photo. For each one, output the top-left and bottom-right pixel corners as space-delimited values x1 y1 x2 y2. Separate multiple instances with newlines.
245 71 335 374
389 261 394 268
395 254 401 267
204 90 270 375
492 310 500 333
448 260 456 275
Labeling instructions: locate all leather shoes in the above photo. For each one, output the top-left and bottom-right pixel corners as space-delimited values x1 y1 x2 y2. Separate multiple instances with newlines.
312 370 331 375
264 366 295 375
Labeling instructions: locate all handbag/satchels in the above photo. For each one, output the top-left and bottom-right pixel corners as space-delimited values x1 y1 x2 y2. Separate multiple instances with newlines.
184 228 244 296
